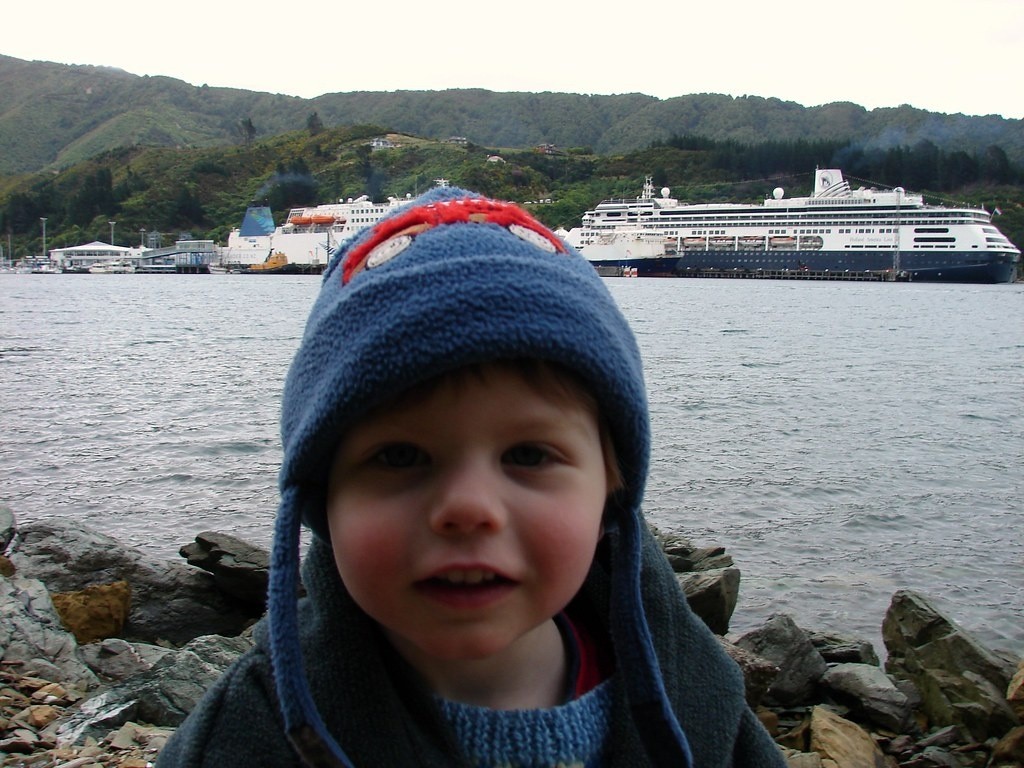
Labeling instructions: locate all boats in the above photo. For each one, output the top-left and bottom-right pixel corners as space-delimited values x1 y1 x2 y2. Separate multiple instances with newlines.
31 259 175 275
220 193 418 275
562 173 1022 284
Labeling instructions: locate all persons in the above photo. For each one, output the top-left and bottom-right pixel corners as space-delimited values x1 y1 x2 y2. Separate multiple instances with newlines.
155 186 788 768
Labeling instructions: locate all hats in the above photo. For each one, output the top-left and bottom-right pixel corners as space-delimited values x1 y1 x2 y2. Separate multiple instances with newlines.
265 186 694 768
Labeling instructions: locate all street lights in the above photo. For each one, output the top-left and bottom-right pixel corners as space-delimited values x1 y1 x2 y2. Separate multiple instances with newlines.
109 221 116 246
140 227 146 247
40 217 48 256
